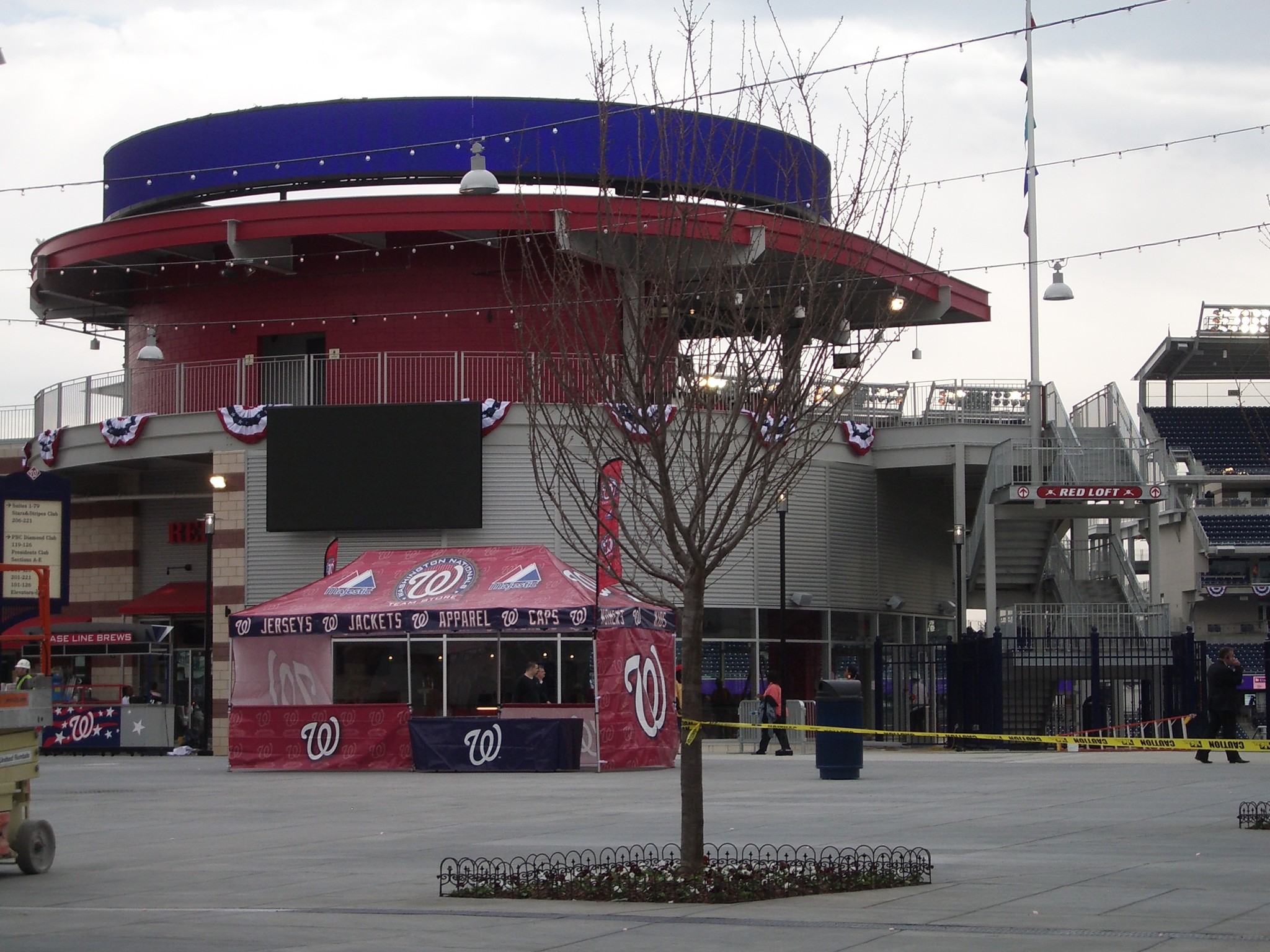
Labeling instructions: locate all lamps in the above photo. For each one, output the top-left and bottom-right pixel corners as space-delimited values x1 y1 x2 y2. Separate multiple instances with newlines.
209 475 231 488
938 600 956 614
794 291 805 318
886 595 906 610
839 302 850 331
225 605 231 617
459 136 500 195
912 325 922 359
137 324 164 362
1043 258 1075 300
888 283 906 312
90 324 100 349
734 291 744 305
790 592 813 606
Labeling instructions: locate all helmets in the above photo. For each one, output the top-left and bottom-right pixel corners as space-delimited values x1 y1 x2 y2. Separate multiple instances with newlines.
912 672 921 679
15 659 31 669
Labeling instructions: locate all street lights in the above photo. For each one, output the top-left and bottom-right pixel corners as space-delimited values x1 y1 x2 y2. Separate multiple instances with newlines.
774 488 790 701
953 523 967 645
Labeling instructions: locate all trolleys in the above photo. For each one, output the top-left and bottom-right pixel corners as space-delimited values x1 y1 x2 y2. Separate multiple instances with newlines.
0 561 56 877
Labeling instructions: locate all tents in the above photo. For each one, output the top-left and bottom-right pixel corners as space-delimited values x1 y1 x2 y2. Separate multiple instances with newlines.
228 544 679 774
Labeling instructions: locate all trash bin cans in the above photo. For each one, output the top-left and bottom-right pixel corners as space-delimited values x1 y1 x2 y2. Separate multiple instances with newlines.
814 679 865 780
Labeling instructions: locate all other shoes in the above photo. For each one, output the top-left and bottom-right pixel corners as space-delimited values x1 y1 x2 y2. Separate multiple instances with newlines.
751 751 766 755
1230 757 1249 764
1195 756 1212 762
775 749 792 755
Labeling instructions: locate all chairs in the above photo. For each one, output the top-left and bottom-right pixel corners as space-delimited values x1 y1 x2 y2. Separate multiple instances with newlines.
1143 407 1270 674
676 641 945 679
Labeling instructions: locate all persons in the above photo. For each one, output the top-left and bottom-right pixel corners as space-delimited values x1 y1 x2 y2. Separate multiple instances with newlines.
845 666 860 680
13 659 34 690
122 684 162 704
1195 646 1250 764
510 662 551 704
911 672 928 704
751 671 792 755
709 677 732 739
